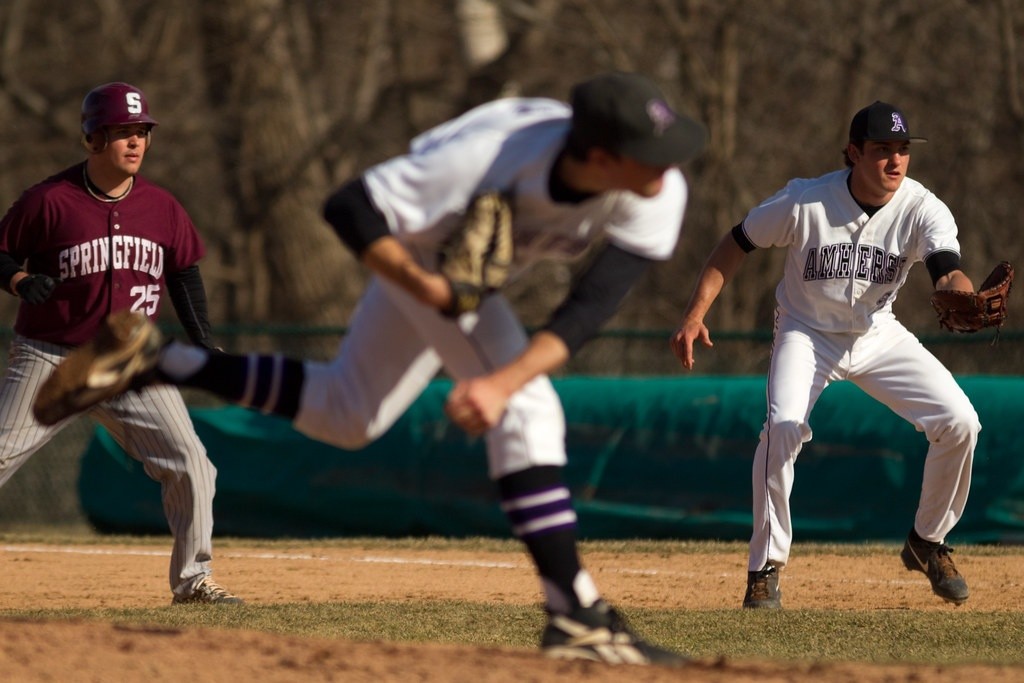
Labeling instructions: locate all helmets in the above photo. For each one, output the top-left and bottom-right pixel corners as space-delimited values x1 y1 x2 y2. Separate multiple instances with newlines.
78 81 160 154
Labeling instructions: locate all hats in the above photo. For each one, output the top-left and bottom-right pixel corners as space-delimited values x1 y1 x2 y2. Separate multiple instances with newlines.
573 74 709 166
849 101 929 145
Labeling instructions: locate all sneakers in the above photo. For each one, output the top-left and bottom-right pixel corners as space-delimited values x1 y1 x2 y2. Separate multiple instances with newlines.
171 579 240 606
542 601 691 668
900 526 969 607
742 563 782 610
33 310 170 427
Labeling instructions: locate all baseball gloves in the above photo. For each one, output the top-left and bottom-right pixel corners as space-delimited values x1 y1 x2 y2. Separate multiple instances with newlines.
931 260 1015 334
442 190 518 321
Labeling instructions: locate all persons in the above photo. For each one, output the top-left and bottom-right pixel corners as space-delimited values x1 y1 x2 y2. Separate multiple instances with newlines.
0 80 250 606
670 100 1013 611
27 69 709 671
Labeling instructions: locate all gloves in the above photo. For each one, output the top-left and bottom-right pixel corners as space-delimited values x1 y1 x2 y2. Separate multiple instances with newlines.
14 274 62 312
